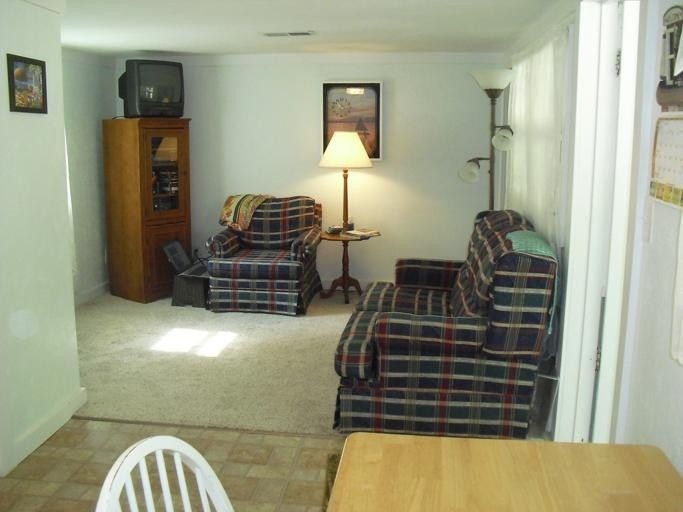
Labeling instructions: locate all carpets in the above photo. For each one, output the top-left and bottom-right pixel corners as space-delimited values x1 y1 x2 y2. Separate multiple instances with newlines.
72 286 364 436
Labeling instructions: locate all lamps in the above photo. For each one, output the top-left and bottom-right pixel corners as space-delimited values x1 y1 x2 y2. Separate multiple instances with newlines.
153 137 178 162
458 68 517 211
318 131 373 234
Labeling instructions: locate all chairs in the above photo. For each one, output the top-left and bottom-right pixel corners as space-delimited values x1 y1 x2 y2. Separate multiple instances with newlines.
94 434 234 512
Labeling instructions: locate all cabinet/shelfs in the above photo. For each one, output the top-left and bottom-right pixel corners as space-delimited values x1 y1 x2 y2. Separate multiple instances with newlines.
102 117 192 304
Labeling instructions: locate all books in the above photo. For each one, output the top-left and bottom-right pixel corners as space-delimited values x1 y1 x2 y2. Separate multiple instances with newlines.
346 229 381 237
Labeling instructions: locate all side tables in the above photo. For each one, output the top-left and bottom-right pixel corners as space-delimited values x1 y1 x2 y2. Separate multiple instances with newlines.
320 229 380 304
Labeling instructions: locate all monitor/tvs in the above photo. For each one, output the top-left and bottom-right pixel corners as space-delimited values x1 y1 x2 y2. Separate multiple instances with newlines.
118 59 185 118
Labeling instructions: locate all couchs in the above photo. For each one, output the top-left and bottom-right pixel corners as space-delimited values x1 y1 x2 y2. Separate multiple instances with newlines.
332 208 559 435
205 193 323 317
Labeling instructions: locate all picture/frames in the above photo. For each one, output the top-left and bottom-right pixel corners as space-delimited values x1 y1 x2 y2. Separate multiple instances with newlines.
320 81 383 162
6 52 48 114
